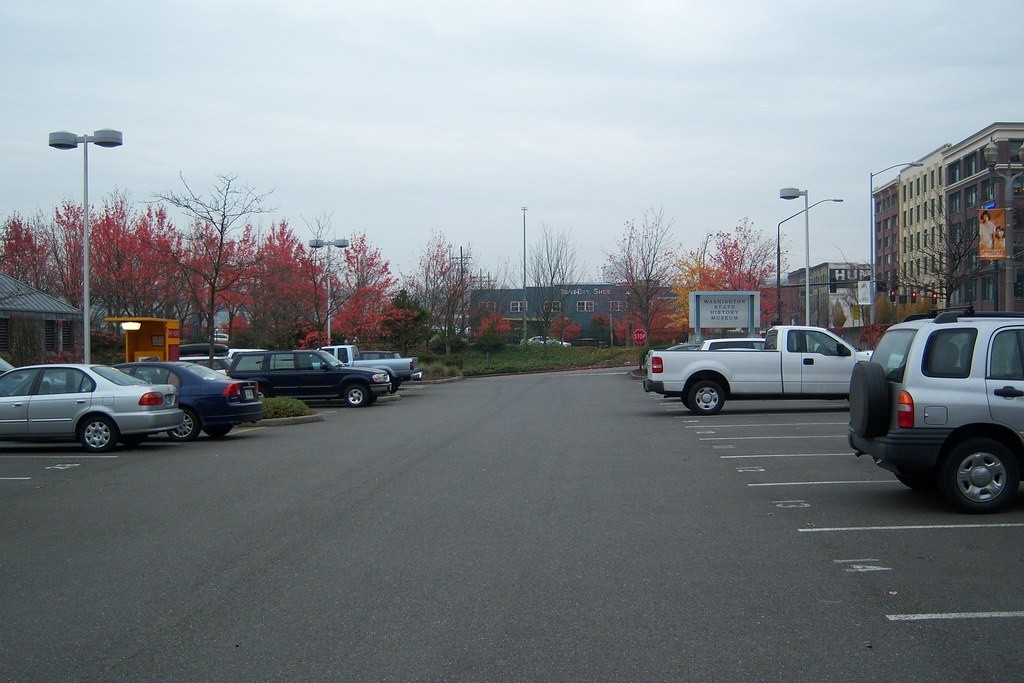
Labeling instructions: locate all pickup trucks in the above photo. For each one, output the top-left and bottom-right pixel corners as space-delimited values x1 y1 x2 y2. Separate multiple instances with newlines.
310 344 423 394
642 323 906 416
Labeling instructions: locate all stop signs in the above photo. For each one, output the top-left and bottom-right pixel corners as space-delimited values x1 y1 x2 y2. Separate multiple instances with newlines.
632 329 648 344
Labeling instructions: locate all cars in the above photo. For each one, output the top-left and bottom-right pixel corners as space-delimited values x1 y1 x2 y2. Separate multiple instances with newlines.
528 335 572 349
177 333 231 376
645 335 767 370
0 362 185 454
0 357 67 386
77 358 264 443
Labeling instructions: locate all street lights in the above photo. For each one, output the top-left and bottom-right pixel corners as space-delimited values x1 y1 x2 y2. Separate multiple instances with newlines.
982 137 1024 310
776 198 845 325
308 238 350 346
520 206 528 344
778 186 812 352
869 161 925 330
47 130 123 364
215 328 218 342
702 233 714 275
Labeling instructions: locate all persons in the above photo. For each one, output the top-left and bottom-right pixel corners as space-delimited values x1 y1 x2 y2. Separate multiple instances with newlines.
979 211 1005 250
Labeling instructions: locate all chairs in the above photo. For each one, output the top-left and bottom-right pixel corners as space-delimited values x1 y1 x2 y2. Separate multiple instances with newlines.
38 381 50 394
151 373 161 384
933 342 969 373
136 372 144 380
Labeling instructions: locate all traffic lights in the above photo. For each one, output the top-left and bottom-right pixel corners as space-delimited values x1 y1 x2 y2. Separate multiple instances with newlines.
932 292 937 305
890 290 896 303
910 291 917 304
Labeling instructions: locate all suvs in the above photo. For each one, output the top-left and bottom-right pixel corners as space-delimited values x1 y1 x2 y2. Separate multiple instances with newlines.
845 304 1024 515
225 349 393 408
